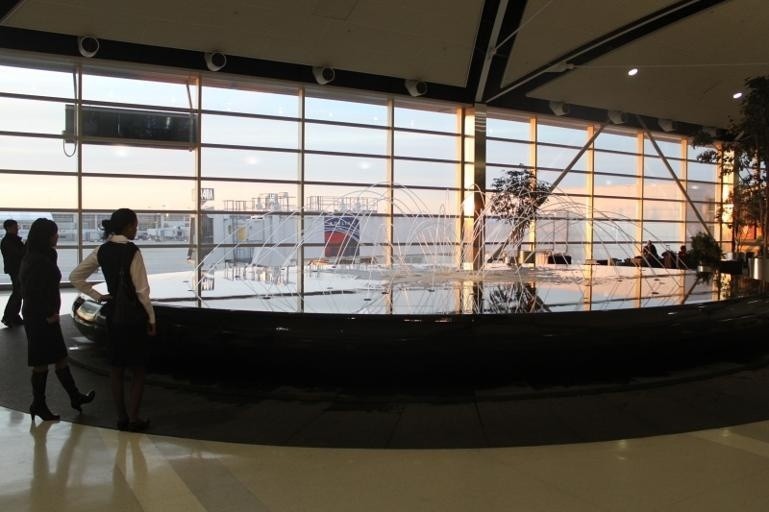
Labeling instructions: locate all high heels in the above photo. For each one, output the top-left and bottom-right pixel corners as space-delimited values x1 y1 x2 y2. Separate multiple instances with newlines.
71 389 94 412
30 404 59 421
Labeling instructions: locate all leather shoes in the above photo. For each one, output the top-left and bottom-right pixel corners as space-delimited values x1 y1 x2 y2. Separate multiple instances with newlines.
118 418 150 430
1 318 23 327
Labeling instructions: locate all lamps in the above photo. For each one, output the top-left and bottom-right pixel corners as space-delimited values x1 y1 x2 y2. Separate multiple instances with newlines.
204 50 227 71
549 101 571 117
312 65 335 85
404 79 428 98
78 32 100 58
657 118 678 132
703 127 720 137
608 110 629 125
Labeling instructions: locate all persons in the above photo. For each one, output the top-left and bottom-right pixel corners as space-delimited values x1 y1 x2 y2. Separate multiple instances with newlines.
678 245 690 269
0 219 25 328
642 240 658 268
68 207 157 430
109 429 147 511
28 416 94 511
17 218 96 421
